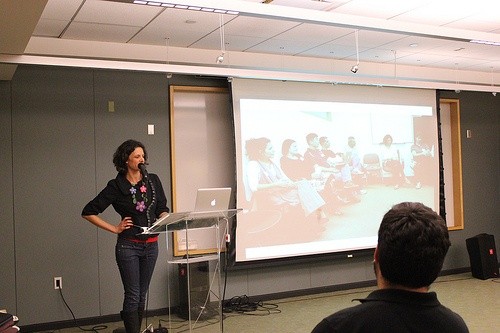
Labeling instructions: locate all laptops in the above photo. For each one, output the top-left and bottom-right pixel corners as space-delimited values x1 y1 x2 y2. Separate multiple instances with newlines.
178 187 232 213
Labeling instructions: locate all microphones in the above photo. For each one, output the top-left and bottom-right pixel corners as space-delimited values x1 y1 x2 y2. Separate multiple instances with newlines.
138 162 149 179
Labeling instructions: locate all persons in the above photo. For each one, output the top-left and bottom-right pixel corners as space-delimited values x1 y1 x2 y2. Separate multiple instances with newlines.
311 203 470 333
81 140 169 333
246 133 438 231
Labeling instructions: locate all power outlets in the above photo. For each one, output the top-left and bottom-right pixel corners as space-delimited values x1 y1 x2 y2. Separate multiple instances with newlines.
54 277 62 290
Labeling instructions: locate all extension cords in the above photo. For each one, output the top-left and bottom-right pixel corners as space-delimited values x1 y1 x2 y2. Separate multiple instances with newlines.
144 327 168 333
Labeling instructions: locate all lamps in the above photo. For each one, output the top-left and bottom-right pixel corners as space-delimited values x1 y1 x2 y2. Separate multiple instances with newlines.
351 25 360 74
216 12 226 64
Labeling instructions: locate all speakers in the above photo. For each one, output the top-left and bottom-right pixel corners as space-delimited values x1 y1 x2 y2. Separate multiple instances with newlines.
465 234 499 280
178 260 210 320
